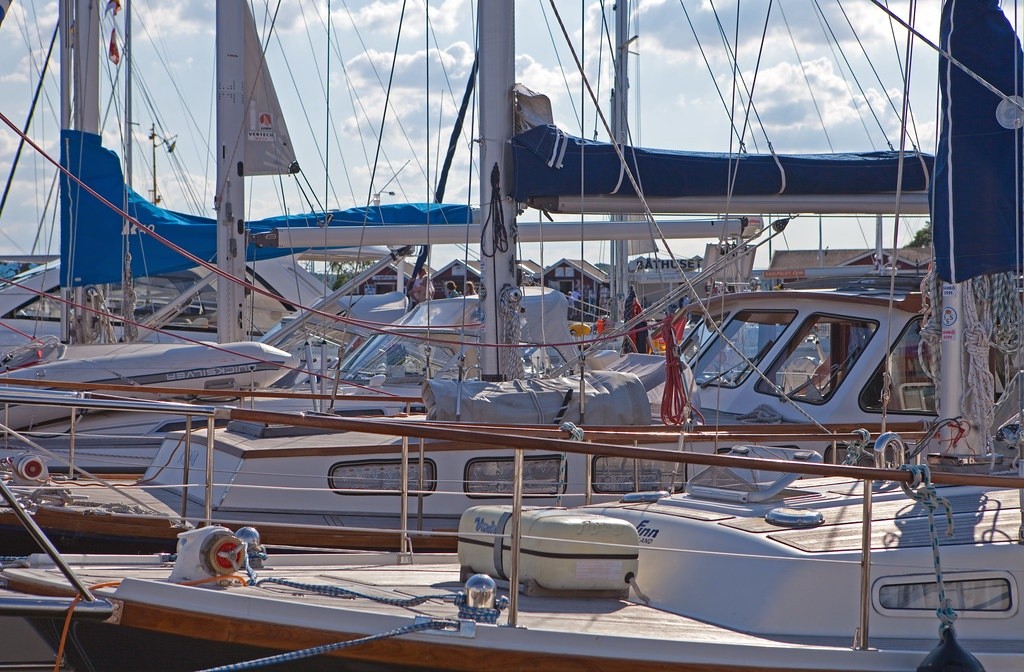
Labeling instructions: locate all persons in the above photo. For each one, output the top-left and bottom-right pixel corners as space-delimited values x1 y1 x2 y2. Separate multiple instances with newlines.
465 282 477 297
446 281 459 299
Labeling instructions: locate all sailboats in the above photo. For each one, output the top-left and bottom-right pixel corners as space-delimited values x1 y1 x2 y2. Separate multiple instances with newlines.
0 2 1024 672
